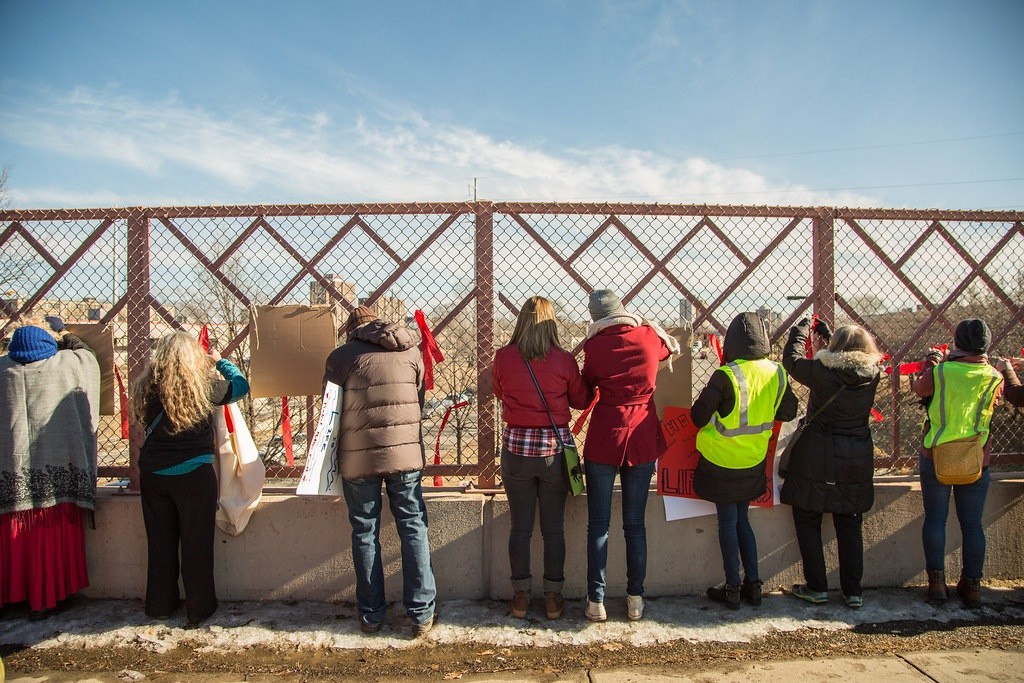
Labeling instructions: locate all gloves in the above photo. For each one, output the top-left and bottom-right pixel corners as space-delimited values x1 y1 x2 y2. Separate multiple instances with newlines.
42 316 65 333
814 318 833 342
798 318 810 328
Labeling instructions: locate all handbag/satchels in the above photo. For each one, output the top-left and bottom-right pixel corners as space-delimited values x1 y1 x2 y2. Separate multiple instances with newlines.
778 416 809 479
563 443 585 498
932 433 984 485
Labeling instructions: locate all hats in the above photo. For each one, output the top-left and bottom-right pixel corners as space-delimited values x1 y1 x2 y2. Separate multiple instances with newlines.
588 289 622 321
954 318 992 354
345 306 379 334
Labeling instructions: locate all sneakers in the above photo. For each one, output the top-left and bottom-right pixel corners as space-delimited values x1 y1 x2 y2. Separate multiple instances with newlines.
792 584 829 603
740 577 763 606
707 584 741 611
584 593 607 621
627 594 645 620
842 594 863 607
544 591 565 619
512 590 531 617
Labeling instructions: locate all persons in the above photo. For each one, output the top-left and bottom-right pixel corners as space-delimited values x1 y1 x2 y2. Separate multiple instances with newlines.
323 305 437 633
995 357 1024 407
915 318 1004 604
782 316 880 607
492 296 594 618
135 332 250 624
581 289 680 621
0 317 101 621
690 312 798 609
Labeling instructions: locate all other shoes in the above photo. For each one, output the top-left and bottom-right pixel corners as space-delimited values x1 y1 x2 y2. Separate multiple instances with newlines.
957 569 983 607
30 597 74 621
928 570 948 599
145 599 185 619
413 612 438 634
